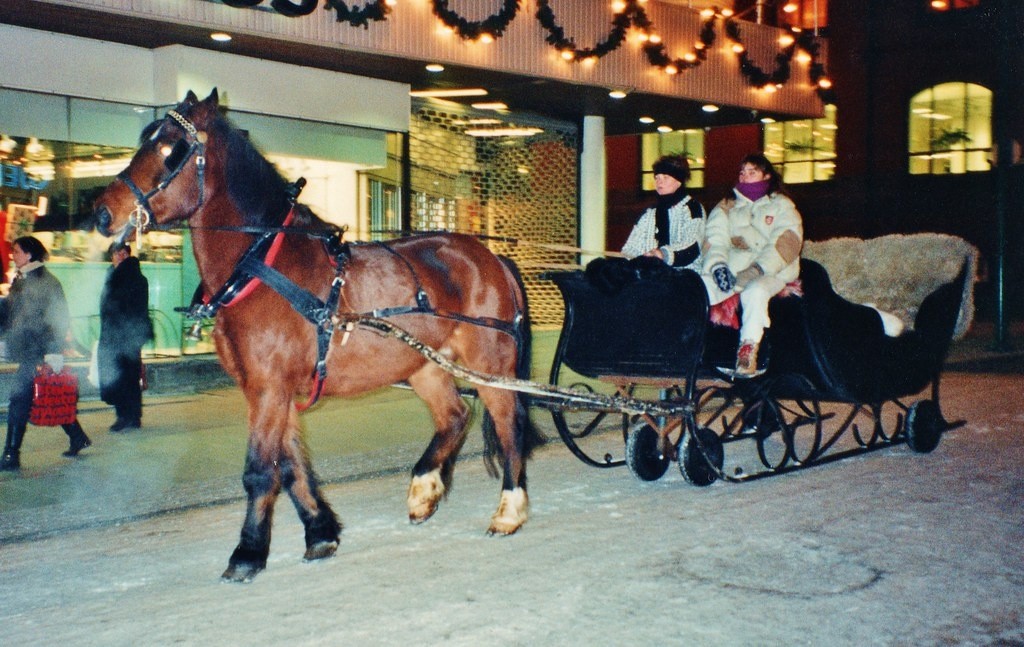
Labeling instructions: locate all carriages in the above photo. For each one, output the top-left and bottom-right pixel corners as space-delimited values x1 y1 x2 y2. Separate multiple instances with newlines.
92 87 979 585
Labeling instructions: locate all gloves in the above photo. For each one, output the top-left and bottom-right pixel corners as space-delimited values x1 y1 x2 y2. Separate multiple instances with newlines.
733 263 764 294
709 262 736 293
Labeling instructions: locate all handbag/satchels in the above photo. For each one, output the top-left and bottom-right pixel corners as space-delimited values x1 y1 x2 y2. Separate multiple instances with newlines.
28 365 79 427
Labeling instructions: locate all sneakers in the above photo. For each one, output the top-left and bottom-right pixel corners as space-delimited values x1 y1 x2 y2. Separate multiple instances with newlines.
734 344 759 375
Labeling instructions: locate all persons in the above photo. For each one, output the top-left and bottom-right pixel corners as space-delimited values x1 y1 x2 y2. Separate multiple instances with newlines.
707 154 803 376
0 236 92 471
621 151 707 276
97 239 155 433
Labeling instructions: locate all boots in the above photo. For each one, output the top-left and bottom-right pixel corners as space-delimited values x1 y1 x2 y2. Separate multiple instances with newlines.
0 396 31 470
60 420 91 458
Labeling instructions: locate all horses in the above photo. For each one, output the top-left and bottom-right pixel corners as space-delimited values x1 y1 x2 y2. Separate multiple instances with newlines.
92 87 551 585
